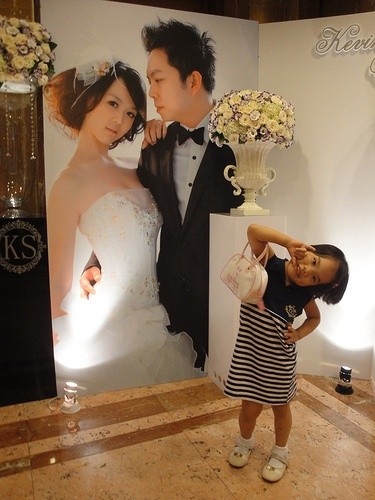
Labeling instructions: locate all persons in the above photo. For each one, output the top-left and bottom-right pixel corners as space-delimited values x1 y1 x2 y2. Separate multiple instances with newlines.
224 223 349 482
80 13 246 371
43 58 205 397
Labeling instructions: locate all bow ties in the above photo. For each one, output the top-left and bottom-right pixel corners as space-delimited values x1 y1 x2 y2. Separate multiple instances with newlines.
177 126 205 145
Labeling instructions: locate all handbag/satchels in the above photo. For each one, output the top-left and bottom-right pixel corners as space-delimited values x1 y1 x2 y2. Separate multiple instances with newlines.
220 242 269 312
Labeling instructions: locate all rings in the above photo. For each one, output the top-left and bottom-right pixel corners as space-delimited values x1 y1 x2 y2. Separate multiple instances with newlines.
157 122 160 126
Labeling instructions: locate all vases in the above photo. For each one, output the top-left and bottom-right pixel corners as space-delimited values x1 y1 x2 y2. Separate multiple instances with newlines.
222 139 277 217
0 72 36 94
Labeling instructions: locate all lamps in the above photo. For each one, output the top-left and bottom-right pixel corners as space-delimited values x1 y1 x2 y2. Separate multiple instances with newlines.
60 381 82 414
334 365 354 396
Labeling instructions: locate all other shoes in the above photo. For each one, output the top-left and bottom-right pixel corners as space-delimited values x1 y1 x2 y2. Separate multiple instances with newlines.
228 444 252 467
262 453 288 482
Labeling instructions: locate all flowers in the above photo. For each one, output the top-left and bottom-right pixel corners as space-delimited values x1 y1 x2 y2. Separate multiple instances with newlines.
0 13 58 88
207 88 297 150
